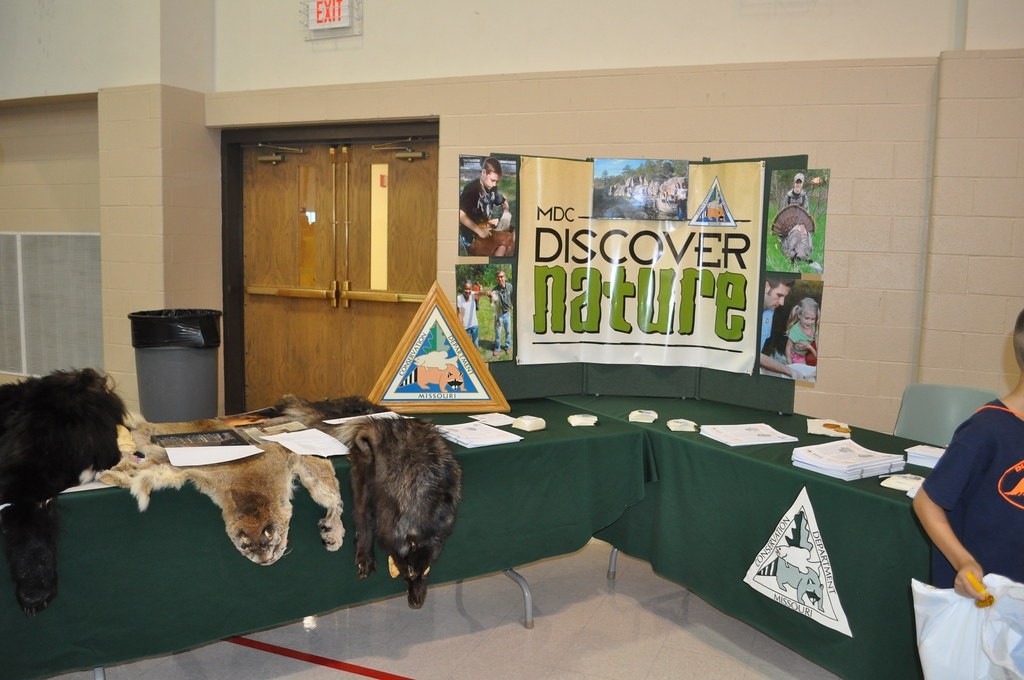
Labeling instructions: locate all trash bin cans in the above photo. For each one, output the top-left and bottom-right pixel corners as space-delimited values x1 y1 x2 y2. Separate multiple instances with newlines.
126 307 223 424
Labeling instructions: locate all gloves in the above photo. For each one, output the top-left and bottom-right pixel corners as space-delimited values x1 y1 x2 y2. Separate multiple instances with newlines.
495 209 514 231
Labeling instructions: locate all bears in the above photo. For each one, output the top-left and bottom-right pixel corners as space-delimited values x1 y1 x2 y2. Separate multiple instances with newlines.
320 394 462 609
0 370 130 620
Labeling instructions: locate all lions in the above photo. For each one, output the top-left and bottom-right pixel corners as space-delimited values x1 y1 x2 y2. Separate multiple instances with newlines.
113 406 345 566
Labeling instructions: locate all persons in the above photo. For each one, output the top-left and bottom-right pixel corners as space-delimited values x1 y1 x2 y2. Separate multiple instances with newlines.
759 276 808 382
460 157 515 257
486 271 514 356
806 370 817 379
781 172 810 213
910 306 1024 607
783 298 821 367
472 281 482 311
456 282 490 349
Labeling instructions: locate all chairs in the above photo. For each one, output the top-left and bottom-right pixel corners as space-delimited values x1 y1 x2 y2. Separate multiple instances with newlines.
892 384 1000 450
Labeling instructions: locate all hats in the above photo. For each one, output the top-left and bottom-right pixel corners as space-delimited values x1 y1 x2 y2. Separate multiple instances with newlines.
794 172 804 183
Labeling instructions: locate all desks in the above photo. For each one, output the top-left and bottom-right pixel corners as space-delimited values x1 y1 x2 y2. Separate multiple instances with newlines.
546 391 947 680
0 396 658 680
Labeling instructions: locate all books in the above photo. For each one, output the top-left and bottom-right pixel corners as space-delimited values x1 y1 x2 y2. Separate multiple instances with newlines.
433 419 526 449
791 437 906 482
904 442 946 470
699 422 800 448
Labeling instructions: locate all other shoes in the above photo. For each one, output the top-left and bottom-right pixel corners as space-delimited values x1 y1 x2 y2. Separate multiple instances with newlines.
506 349 512 355
494 349 499 356
